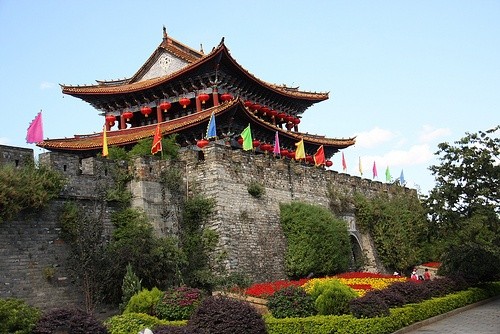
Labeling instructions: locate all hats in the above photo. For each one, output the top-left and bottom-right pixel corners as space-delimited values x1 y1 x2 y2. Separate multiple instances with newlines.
412 272 416 274
393 272 398 276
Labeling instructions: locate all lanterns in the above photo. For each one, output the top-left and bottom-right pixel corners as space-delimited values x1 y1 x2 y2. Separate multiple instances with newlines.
221 93 233 102
179 98 190 108
197 140 209 153
238 138 244 144
326 161 333 167
123 111 133 121
306 155 315 163
160 102 171 112
253 139 295 160
141 106 152 117
106 114 116 125
245 100 300 125
198 93 209 104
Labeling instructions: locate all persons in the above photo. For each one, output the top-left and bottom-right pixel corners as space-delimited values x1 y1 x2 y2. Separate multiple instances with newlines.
393 267 430 281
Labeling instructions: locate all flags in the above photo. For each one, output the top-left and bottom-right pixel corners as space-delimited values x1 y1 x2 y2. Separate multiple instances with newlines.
360 159 363 175
205 114 215 140
102 126 108 156
373 164 377 179
385 168 391 180
241 126 253 151
314 146 325 167
295 139 306 159
342 155 347 169
151 124 162 155
273 134 280 154
401 170 404 183
27 113 43 143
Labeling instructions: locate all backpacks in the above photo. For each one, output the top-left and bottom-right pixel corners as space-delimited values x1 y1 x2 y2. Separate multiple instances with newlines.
412 275 417 280
